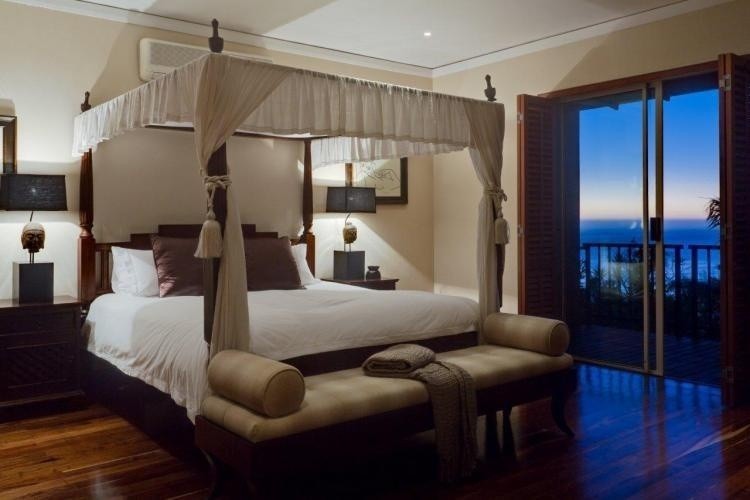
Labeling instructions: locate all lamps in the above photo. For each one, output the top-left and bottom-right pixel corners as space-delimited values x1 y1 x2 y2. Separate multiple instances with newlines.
1 174 69 303
325 187 376 279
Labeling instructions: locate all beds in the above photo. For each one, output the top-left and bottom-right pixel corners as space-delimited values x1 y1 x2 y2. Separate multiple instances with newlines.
78 222 503 480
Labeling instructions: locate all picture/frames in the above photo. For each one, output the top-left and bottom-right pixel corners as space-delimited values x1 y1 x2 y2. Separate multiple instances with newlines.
344 156 409 205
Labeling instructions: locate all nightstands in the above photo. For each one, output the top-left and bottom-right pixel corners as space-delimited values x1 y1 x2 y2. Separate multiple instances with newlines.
321 277 400 290
0 295 86 424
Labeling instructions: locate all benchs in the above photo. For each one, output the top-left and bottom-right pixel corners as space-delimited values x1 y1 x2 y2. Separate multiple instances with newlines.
193 312 579 498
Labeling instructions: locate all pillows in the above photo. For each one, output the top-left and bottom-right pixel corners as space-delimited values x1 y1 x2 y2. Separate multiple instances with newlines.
107 234 316 297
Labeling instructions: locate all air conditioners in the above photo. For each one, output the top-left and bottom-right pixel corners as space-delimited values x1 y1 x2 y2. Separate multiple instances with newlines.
139 37 276 86
